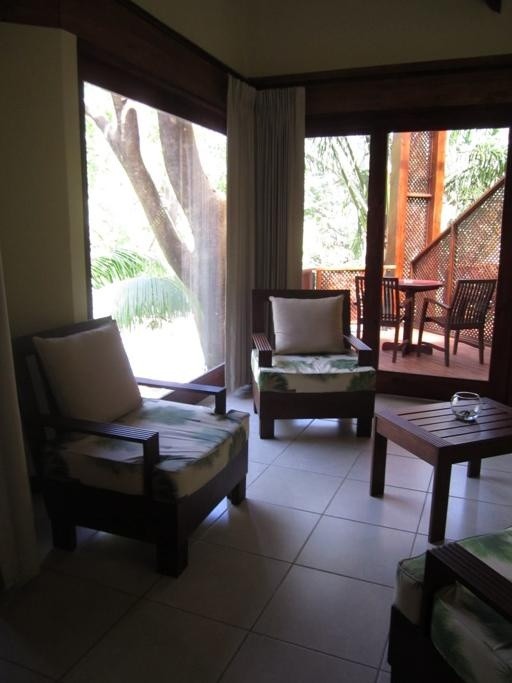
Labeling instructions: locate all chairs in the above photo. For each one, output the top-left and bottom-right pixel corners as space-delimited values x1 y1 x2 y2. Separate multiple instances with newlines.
355 275 407 361
250 288 376 440
11 316 250 577
389 524 512 683
418 278 496 367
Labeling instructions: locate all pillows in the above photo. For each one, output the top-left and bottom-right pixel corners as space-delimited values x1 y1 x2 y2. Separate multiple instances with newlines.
267 293 349 356
31 320 143 442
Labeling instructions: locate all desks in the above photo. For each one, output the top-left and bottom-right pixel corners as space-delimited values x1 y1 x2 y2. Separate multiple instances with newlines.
381 279 443 357
